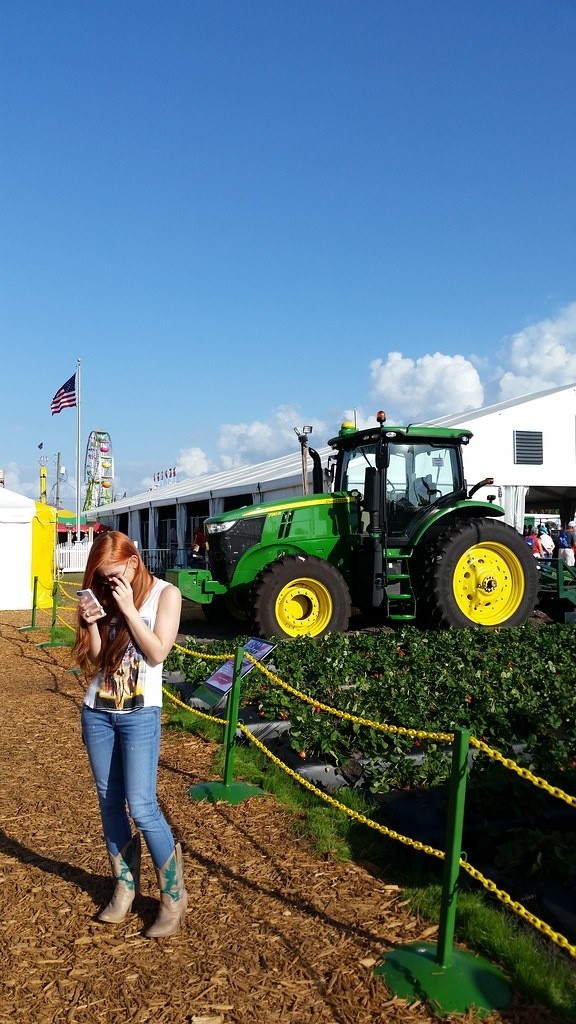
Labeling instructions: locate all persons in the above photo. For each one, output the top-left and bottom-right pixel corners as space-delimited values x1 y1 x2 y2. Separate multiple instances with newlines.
522 521 576 575
69 529 191 940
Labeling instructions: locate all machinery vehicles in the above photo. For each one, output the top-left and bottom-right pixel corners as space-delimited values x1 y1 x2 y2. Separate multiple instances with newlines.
166 411 576 646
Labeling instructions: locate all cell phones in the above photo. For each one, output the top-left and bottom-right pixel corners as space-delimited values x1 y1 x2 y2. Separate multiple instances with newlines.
76 589 104 616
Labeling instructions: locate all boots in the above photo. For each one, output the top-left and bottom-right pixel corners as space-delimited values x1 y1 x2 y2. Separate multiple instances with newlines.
97 831 143 923
146 843 189 938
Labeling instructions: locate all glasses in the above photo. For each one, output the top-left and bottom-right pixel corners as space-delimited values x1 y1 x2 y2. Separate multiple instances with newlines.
98 557 130 589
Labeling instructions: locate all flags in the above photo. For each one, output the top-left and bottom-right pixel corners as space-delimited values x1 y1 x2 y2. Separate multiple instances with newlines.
50 373 78 416
37 442 44 451
